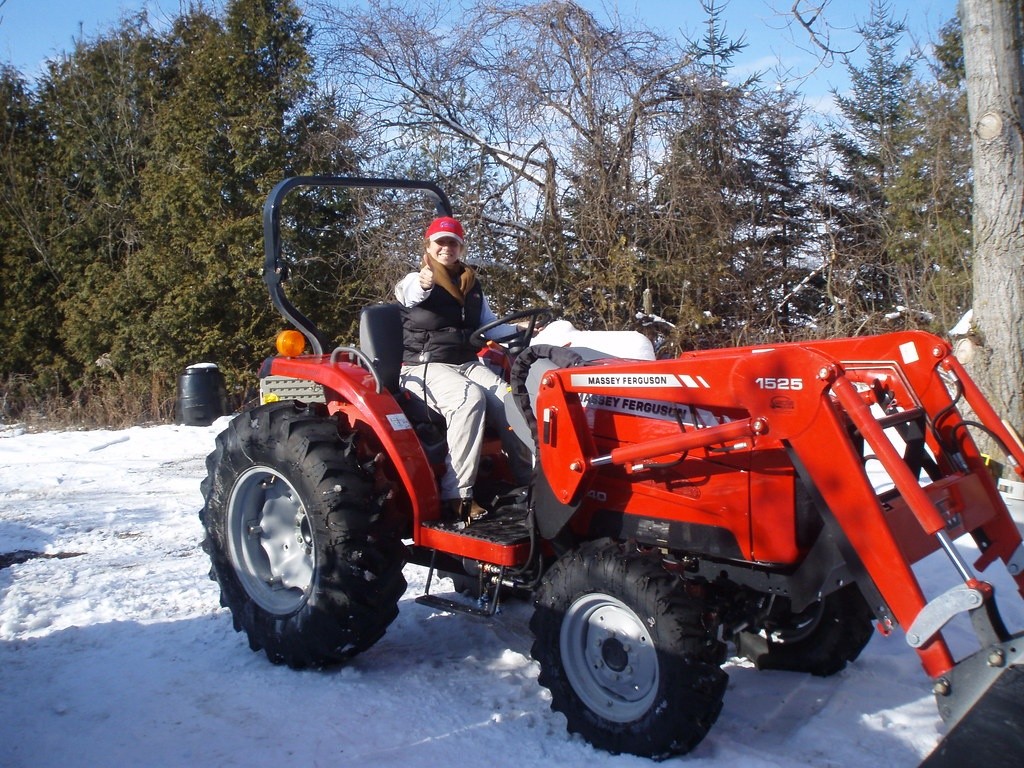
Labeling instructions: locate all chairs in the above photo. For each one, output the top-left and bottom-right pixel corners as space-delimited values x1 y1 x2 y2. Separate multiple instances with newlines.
357 305 402 390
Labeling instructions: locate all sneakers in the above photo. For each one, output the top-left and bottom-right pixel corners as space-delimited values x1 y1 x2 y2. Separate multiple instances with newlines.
442 498 488 519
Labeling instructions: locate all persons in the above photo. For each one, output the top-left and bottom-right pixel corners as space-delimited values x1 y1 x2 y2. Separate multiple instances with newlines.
394 218 543 520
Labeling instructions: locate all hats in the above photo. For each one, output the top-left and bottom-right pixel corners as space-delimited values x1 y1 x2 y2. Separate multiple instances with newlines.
425 217 464 245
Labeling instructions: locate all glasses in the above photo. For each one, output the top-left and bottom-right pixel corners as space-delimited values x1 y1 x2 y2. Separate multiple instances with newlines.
434 240 458 247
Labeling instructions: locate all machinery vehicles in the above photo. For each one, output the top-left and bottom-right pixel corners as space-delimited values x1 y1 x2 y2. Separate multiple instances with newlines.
178 174 1023 768
